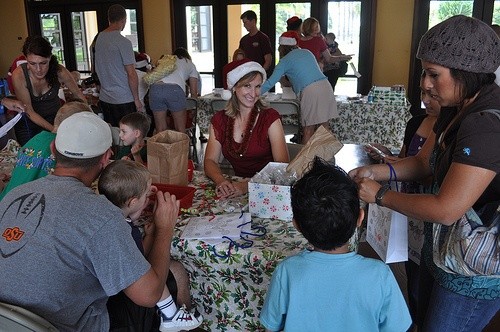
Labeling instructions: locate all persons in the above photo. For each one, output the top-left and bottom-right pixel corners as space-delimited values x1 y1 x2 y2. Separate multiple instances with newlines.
348 16 500 332
255 166 413 332
222 8 355 96
260 30 337 167
366 68 453 161
203 59 293 201
98 161 204 332
87 5 202 134
0 101 95 201
0 33 94 150
111 117 156 168
0 111 179 332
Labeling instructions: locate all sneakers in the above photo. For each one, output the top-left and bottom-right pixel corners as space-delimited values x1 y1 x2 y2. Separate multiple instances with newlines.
159 303 204 332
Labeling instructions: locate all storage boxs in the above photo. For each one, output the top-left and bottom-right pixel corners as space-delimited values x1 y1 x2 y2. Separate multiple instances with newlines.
247 162 295 223
372 87 408 107
145 182 197 217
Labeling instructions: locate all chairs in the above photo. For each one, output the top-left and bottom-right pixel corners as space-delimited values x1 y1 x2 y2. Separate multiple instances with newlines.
212 98 302 144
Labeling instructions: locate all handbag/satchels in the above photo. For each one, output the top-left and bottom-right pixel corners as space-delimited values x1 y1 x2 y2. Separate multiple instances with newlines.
147 129 189 187
432 108 500 277
142 54 177 86
366 163 409 264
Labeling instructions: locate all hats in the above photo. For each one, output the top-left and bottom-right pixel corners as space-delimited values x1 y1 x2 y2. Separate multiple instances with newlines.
280 31 303 50
287 16 302 30
220 57 268 100
416 14 500 73
54 111 112 159
135 52 152 70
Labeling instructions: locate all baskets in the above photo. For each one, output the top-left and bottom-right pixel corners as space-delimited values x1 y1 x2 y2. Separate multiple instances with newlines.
149 182 197 212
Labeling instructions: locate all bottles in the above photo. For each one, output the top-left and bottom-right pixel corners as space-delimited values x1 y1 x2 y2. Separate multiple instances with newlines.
0 77 6 115
3 76 9 96
86 89 93 106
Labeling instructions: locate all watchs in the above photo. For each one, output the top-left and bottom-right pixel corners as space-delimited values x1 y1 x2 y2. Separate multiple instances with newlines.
375 182 392 206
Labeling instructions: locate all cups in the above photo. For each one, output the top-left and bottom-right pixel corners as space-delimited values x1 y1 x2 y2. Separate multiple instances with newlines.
212 88 224 98
220 89 232 100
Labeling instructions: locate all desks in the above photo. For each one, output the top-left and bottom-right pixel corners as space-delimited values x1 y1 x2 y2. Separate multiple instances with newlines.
63 84 100 107
196 93 412 156
0 149 360 332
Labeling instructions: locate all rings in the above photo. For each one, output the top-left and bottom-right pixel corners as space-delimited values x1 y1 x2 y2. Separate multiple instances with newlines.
217 186 222 189
